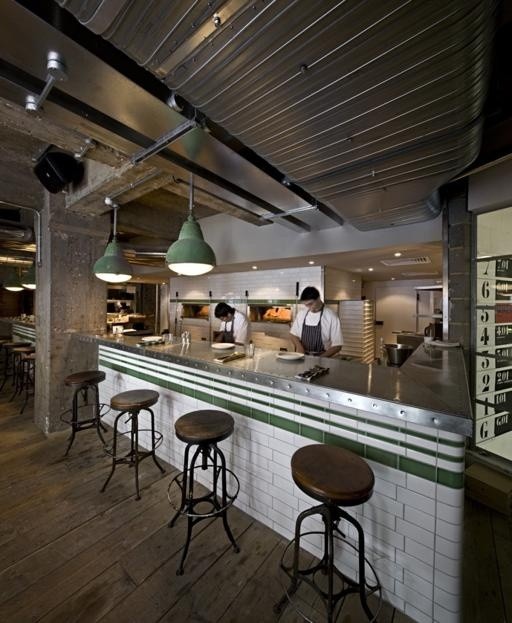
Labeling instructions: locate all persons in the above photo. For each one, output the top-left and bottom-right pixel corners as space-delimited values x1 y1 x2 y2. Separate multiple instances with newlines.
214 302 251 346
289 286 344 358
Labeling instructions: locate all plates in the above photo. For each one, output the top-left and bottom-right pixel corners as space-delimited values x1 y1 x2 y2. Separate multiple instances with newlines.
141 336 163 341
211 343 236 349
277 352 304 360
123 328 136 333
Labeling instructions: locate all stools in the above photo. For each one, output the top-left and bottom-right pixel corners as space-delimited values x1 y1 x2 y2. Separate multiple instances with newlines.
60 370 111 457
99 389 165 500
272 443 382 623
20 355 40 415
0 341 31 392
166 408 241 576
8 346 35 403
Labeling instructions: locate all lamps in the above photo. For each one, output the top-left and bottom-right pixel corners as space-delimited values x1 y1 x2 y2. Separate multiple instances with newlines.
93 203 134 283
165 172 216 277
20 261 37 289
3 270 25 291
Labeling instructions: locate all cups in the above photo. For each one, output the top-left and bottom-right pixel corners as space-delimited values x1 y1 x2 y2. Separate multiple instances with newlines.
247 344 255 357
186 330 192 344
113 325 123 335
181 331 186 345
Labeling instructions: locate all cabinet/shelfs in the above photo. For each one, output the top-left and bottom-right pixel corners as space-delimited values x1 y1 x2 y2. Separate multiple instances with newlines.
414 282 441 336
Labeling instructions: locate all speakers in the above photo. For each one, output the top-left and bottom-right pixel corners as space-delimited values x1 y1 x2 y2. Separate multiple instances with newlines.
32 143 80 194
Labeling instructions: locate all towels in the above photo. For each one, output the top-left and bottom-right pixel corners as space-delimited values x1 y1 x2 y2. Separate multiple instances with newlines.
213 351 244 362
136 338 165 348
295 364 330 383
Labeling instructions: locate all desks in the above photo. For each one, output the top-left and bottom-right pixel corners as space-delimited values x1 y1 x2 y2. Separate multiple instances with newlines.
465 459 512 525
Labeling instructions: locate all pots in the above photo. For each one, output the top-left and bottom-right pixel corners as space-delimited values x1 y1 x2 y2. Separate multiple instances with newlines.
383 343 413 365
